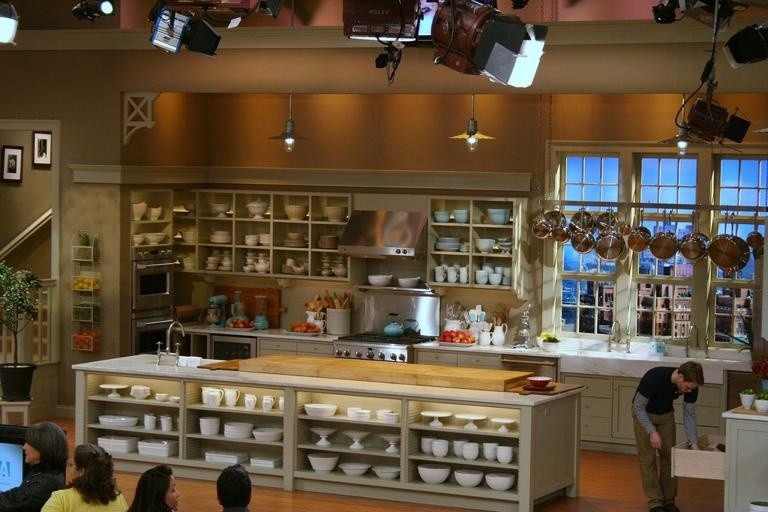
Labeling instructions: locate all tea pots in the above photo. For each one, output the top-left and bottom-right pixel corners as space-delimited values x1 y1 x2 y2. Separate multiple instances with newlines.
403 319 421 336
383 313 404 336
443 318 480 339
320 253 347 277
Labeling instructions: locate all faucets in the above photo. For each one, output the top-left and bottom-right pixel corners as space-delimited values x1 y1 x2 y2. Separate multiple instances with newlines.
157 321 186 366
607 320 631 354
686 325 708 360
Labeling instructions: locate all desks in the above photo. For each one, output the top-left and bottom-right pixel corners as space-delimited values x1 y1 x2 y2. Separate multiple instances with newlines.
71 353 588 407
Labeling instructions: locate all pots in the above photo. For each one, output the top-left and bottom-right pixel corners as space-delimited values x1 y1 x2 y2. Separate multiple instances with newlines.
246 198 269 218
620 206 631 235
570 205 595 236
552 200 572 244
709 207 749 273
594 204 625 262
635 204 651 237
545 211 565 231
651 206 679 259
747 207 765 248
679 206 710 260
532 199 552 240
627 206 651 252
596 203 620 233
570 203 595 255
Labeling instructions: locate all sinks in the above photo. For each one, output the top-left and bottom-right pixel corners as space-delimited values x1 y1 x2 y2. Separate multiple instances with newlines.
144 357 226 368
560 350 747 384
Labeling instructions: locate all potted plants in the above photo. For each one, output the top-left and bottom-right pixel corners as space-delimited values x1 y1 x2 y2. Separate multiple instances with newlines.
0 261 41 400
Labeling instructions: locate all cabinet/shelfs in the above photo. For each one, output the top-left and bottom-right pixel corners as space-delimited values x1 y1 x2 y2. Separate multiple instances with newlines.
414 349 557 384
355 193 441 297
611 358 668 457
284 387 401 502
427 170 533 301
56 165 175 418
401 394 581 512
670 406 768 512
559 357 611 453
257 338 334 358
71 228 101 353
176 332 257 362
667 357 727 464
175 166 369 285
74 370 185 479
183 379 284 489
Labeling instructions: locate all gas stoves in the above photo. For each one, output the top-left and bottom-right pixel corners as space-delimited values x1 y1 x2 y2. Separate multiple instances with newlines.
331 333 436 363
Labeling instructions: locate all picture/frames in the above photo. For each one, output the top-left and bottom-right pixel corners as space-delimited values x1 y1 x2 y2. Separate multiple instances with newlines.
1 145 24 183
32 131 52 166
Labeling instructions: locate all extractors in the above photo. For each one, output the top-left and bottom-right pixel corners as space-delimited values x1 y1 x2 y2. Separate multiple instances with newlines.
338 209 428 256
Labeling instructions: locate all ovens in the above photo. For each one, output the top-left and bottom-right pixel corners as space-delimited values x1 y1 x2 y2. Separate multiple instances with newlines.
129 246 181 355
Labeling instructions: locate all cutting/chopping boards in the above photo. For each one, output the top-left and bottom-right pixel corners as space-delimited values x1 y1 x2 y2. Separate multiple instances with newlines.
197 353 587 397
212 286 287 329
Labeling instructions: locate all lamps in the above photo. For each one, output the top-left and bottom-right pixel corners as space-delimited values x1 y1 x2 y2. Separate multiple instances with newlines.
688 99 751 144
342 0 420 42
0 1 19 46
658 119 710 157
431 1 549 88
72 1 114 23
724 24 767 70
269 118 312 154
147 0 221 57
653 0 748 31
449 117 496 153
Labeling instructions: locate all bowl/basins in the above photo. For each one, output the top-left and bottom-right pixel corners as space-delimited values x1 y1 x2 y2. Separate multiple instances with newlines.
474 239 495 254
181 232 196 243
130 202 148 221
739 394 755 409
503 267 512 285
481 263 504 273
129 235 144 246
397 276 421 288
199 416 220 435
204 249 232 271
454 209 469 223
372 466 401 481
252 427 284 442
208 203 230 217
486 473 516 491
376 409 399 423
755 399 768 412
285 204 308 221
324 207 348 222
489 273 502 285
303 403 338 417
143 413 156 430
487 209 511 224
526 376 552 389
475 270 488 285
308 453 340 474
347 406 371 420
138 232 165 244
454 469 483 488
434 210 451 223
496 238 512 254
160 415 173 432
155 393 167 401
144 206 162 220
169 396 180 403
337 463 371 477
242 249 270 274
418 463 451 484
368 274 393 287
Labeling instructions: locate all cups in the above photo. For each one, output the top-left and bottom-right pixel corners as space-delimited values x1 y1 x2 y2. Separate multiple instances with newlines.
224 389 240 407
496 445 513 465
208 389 224 407
452 439 469 459
461 442 479 461
421 436 437 455
478 331 494 345
459 265 469 283
279 396 285 412
244 393 257 410
262 395 276 411
482 442 499 461
435 263 448 282
447 264 459 283
491 323 508 346
200 386 212 404
432 439 448 458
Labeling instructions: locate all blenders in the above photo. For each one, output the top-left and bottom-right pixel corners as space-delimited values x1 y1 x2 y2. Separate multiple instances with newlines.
253 296 270 330
227 290 249 323
206 295 228 328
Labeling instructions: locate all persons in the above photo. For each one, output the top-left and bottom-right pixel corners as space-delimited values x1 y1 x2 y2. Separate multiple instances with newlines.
631 361 706 511
39 444 130 512
128 464 180 512
1 422 68 511
215 464 252 512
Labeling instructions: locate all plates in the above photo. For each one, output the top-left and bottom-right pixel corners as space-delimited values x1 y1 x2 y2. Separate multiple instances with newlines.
379 434 401 453
223 422 254 439
420 410 452 427
99 384 128 397
97 435 140 453
226 327 254 332
284 232 307 247
318 235 339 248
454 413 487 430
490 418 515 432
244 234 258 245
210 230 231 244
250 457 282 469
437 341 477 347
259 234 270 246
129 384 151 400
435 237 469 252
97 415 138 427
523 385 556 391
281 329 320 337
342 429 371 449
309 427 337 446
205 450 249 465
138 439 180 457
